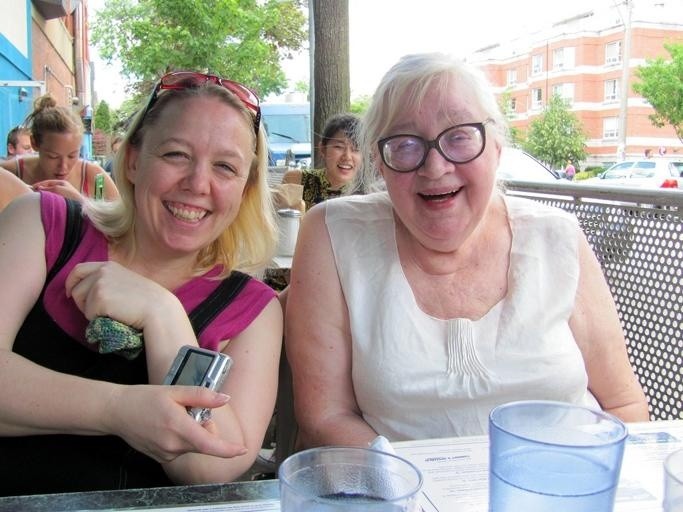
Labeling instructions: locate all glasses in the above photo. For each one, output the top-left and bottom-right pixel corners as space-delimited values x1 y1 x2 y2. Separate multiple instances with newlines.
377 117 492 172
143 70 260 136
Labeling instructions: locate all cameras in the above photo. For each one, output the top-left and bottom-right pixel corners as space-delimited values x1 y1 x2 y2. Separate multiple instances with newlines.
161 345 231 426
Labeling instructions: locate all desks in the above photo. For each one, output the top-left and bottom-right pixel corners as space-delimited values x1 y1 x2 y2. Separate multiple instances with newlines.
0 480 282 512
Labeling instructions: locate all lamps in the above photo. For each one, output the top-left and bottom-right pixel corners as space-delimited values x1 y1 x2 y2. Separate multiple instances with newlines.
64 85 79 105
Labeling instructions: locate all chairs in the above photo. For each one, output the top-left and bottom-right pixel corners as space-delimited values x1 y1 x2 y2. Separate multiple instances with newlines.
275 286 304 478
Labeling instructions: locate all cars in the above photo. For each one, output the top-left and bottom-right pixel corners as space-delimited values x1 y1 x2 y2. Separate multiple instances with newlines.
579 159 683 191
260 101 313 171
496 148 577 186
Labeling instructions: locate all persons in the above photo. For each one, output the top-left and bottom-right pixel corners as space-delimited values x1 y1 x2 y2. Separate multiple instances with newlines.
644 149 654 159
1 71 285 498
565 160 575 181
285 53 649 449
281 112 371 215
2 92 124 210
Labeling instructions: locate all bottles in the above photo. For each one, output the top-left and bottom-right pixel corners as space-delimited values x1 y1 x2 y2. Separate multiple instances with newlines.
93 174 104 202
276 206 302 258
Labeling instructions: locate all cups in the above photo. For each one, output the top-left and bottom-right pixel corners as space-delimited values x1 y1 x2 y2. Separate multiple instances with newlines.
487 399 628 511
664 450 683 511
276 445 424 512
